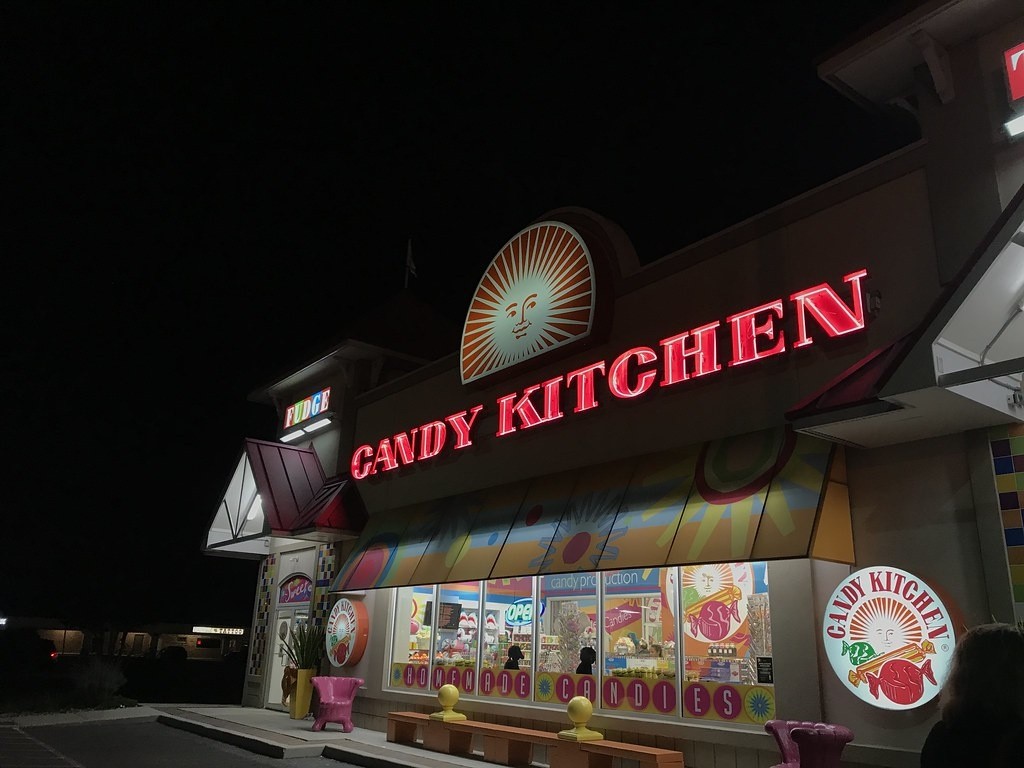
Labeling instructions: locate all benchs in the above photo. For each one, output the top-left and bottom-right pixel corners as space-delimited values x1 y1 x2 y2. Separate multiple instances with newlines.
385 711 684 768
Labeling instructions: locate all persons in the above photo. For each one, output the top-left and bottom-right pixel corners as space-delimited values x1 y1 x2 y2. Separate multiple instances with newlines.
504 646 524 670
576 647 596 674
921 623 1024 768
649 644 663 657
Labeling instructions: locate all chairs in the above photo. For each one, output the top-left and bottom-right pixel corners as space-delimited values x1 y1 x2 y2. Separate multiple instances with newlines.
762 718 854 768
310 676 365 734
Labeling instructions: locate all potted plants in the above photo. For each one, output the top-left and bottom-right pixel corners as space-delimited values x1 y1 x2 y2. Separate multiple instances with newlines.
276 623 327 719
